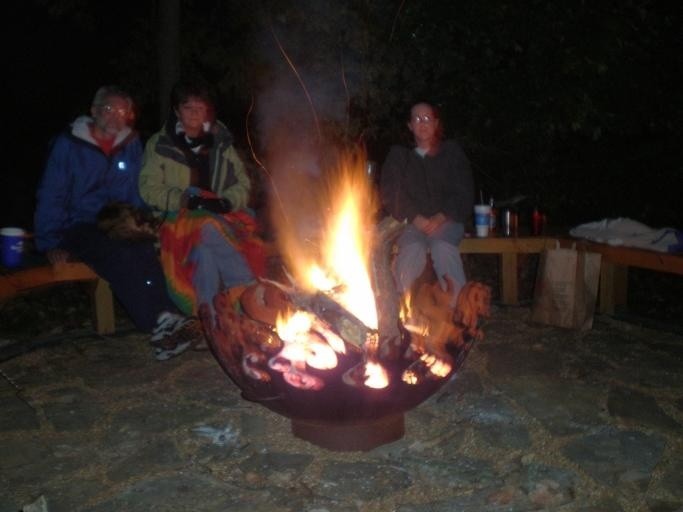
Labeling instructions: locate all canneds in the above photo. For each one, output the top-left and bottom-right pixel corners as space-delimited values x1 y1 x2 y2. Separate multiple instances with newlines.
498 209 511 237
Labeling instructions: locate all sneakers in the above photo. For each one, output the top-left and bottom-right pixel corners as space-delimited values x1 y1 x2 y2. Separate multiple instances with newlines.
150 309 202 361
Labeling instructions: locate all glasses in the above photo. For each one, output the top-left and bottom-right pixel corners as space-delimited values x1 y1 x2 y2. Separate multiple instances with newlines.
102 104 129 119
413 115 429 125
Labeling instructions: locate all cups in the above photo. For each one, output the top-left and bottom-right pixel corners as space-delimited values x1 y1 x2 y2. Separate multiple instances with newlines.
475 205 490 239
1 226 24 271
502 204 519 237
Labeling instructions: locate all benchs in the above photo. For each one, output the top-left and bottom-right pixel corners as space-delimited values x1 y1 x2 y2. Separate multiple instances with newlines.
2 247 118 334
390 223 682 315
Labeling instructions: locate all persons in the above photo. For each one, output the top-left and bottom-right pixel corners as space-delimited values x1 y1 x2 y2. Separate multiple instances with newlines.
136 86 262 321
375 95 475 306
33 84 209 360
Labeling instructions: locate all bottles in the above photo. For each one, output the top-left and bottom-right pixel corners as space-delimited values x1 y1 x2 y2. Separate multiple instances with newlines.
489 199 497 235
